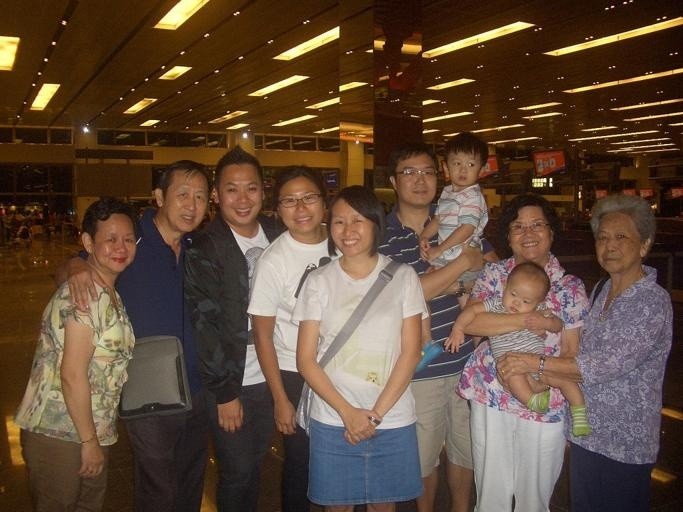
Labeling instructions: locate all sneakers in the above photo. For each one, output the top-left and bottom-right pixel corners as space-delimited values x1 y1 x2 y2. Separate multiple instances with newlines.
414 339 443 374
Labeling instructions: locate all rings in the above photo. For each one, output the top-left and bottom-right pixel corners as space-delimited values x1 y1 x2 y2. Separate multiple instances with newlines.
501 368 505 374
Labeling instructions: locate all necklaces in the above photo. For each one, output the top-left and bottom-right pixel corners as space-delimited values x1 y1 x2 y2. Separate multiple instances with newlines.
600 269 645 321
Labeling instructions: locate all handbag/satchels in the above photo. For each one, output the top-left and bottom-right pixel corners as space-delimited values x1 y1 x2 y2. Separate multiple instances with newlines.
118 336 193 421
295 380 313 435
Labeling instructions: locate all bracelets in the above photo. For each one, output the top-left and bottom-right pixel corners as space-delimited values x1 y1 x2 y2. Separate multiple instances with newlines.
536 353 546 374
80 433 97 445
368 416 380 425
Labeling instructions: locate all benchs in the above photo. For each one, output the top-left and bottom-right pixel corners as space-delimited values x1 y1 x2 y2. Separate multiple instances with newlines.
486 210 683 296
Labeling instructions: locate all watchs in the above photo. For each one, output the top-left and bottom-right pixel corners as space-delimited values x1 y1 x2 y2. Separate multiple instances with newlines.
455 280 466 297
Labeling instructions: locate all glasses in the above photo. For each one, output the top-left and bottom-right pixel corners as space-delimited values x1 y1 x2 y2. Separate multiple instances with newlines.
276 194 323 208
395 169 439 179
507 223 549 235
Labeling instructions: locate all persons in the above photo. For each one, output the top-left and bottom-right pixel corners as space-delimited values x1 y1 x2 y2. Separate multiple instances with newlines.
454 192 592 511
495 195 674 510
16 198 137 509
247 164 342 510
376 142 500 511
183 143 311 511
55 160 208 510
291 185 429 511
416 133 488 374
1 206 69 248
444 262 590 437
